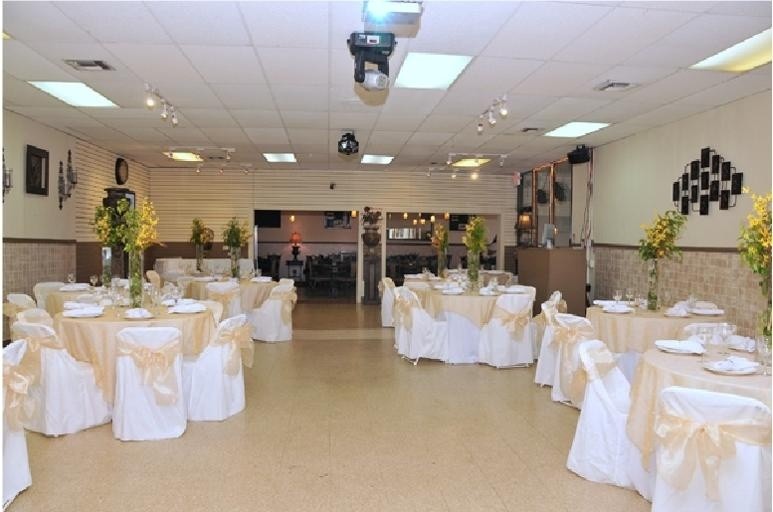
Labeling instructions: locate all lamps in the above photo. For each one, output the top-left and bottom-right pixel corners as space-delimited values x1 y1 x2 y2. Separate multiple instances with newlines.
289 232 302 260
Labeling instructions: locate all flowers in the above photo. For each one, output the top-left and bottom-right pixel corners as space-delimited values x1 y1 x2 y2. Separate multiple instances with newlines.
88 193 168 309
187 215 254 278
430 224 448 277
461 215 490 282
634 207 688 311
734 185 771 350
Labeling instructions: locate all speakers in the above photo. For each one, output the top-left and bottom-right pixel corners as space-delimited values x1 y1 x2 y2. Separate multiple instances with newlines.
568 144 590 164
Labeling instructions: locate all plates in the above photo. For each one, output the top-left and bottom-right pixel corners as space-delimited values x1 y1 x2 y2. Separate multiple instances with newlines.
654 336 761 378
418 276 529 299
62 273 273 321
596 296 722 321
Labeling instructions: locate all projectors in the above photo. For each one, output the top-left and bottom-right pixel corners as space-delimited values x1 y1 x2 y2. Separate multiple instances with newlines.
362 0 422 24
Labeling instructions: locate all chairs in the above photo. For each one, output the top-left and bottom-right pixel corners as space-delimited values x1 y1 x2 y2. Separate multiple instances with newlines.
257 254 281 282
303 255 355 298
386 254 451 287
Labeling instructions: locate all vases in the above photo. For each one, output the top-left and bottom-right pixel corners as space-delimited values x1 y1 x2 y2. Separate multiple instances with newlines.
646 259 659 312
